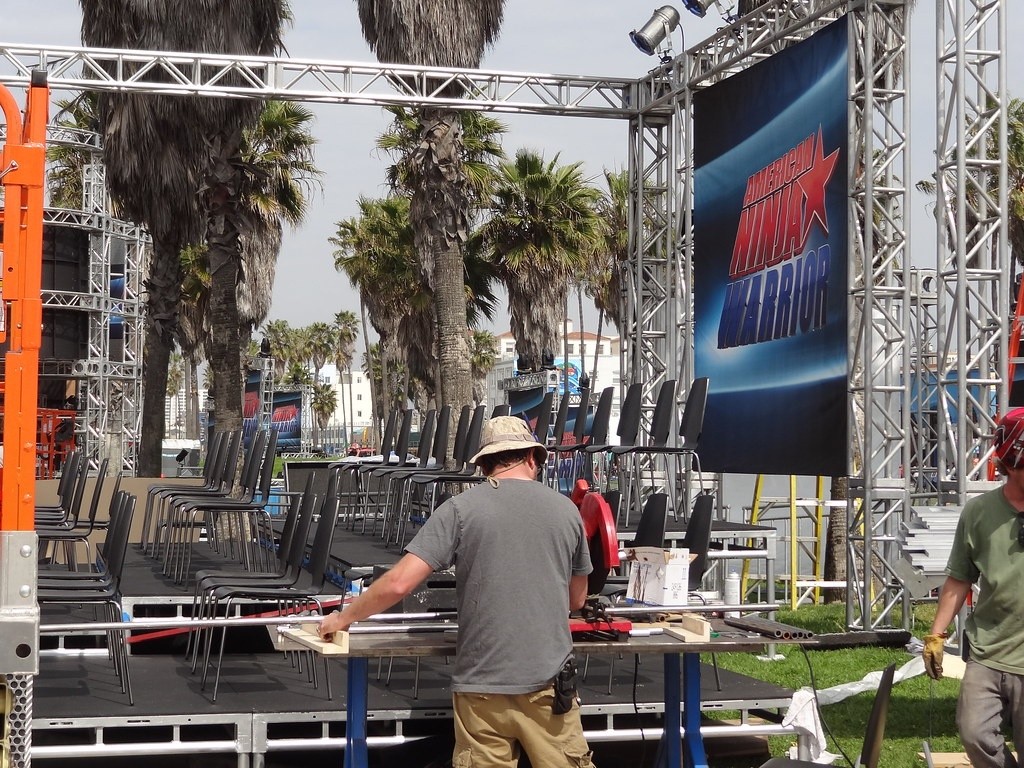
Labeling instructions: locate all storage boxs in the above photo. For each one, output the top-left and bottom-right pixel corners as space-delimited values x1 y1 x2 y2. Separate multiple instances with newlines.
625 547 699 607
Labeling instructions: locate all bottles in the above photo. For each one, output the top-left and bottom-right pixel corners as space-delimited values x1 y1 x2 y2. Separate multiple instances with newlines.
725 569 741 619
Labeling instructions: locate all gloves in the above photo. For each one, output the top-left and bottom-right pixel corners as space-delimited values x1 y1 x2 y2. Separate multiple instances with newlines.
922 632 943 679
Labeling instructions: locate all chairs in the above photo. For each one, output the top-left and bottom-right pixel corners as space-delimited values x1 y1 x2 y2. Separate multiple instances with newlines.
33 375 713 707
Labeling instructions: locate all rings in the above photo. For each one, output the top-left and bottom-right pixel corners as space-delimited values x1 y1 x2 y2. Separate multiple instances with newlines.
316 626 321 633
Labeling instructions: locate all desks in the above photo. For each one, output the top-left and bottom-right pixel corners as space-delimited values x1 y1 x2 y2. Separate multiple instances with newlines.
280 620 816 768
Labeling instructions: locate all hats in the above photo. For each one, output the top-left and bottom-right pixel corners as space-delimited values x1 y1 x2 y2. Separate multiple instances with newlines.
469 416 548 465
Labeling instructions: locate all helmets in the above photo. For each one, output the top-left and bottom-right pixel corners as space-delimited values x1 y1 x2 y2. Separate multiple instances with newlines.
994 407 1024 476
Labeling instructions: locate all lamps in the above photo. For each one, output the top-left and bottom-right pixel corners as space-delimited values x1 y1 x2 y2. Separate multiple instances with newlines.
683 0 739 24
629 5 684 62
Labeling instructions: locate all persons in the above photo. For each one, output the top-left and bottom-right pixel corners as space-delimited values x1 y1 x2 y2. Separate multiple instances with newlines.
922 407 1024 768
316 416 598 768
